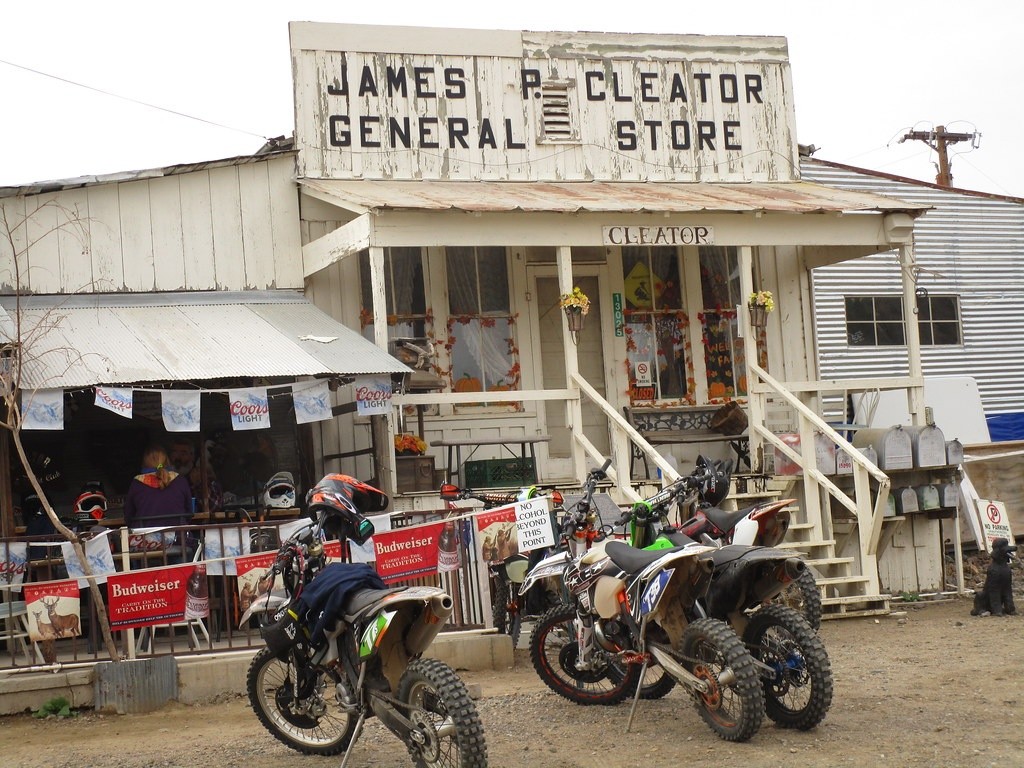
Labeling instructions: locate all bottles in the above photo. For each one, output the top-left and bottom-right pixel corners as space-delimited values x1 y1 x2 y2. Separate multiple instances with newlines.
185 564 209 621
437 522 459 573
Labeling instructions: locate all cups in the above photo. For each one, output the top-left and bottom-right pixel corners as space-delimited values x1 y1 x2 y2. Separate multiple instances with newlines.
192 498 196 513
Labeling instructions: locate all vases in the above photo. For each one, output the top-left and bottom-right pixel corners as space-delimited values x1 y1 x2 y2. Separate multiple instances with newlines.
566 314 585 331
394 449 419 456
749 307 769 327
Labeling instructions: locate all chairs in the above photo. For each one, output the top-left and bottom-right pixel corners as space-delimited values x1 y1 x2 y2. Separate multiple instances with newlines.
830 386 881 436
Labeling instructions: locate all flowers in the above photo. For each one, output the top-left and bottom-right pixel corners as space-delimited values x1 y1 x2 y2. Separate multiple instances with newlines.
394 432 430 453
558 286 592 317
748 289 776 314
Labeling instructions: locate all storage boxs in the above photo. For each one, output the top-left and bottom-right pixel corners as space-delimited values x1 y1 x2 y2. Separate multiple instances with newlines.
464 457 536 488
395 455 437 494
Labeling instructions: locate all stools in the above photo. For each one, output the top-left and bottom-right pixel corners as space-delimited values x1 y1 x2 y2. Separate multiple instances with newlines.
135 615 215 655
132 545 195 570
0 601 46 666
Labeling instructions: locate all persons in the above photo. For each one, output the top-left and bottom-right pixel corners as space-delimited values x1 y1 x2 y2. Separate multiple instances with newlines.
124 438 225 569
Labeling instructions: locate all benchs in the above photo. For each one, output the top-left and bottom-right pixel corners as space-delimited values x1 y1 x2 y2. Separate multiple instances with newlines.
623 405 752 481
429 434 553 489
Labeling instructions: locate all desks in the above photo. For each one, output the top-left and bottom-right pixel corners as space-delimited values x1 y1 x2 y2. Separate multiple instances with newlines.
26 550 170 654
97 507 301 631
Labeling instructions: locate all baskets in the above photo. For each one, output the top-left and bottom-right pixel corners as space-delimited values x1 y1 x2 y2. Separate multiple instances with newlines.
710 401 749 436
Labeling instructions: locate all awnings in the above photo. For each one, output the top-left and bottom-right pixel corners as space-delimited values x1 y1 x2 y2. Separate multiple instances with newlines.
0 287 414 393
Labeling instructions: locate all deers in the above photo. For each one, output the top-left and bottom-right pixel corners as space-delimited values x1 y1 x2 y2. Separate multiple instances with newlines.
32 595 81 639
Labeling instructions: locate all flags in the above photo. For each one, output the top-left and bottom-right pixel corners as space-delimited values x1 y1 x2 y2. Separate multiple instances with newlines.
22 377 396 433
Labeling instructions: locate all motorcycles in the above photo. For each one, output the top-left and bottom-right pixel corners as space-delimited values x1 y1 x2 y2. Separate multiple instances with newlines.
440 482 521 651
238 498 490 768
517 456 834 742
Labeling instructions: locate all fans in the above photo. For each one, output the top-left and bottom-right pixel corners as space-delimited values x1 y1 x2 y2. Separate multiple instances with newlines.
210 427 280 505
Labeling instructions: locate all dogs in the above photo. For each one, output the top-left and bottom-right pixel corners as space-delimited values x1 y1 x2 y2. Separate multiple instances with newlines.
969 537 1019 617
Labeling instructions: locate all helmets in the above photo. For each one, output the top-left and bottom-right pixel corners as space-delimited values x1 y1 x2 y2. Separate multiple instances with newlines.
305 472 389 546
263 471 296 508
72 487 108 519
695 454 733 508
